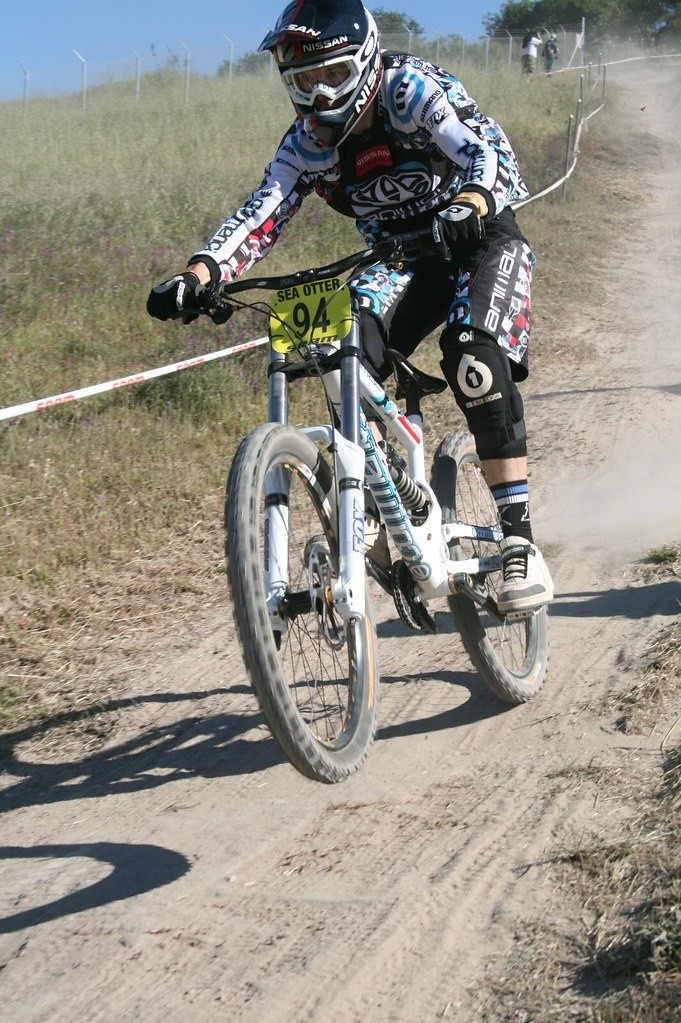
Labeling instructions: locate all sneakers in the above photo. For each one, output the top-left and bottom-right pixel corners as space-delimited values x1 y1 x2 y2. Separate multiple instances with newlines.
498 535 553 612
304 528 390 575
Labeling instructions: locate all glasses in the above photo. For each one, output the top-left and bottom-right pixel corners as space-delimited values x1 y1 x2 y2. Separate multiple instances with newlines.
282 55 361 107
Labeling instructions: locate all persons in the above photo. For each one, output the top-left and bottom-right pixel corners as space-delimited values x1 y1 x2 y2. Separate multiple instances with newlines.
522 31 542 80
541 34 558 75
146 0 555 610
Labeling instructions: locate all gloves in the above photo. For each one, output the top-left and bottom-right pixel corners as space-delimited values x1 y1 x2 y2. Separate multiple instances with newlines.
432 201 482 264
147 271 202 324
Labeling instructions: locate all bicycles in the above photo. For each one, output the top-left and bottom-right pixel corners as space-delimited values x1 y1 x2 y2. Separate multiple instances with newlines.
183 217 551 787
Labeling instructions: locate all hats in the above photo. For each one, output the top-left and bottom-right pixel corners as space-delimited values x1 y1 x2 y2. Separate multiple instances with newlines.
551 34 557 42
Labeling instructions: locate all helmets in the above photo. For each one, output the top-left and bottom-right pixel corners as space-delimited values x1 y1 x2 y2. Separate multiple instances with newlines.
258 0 380 146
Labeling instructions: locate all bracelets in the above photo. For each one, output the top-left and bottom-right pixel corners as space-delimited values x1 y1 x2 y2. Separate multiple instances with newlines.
453 194 484 216
